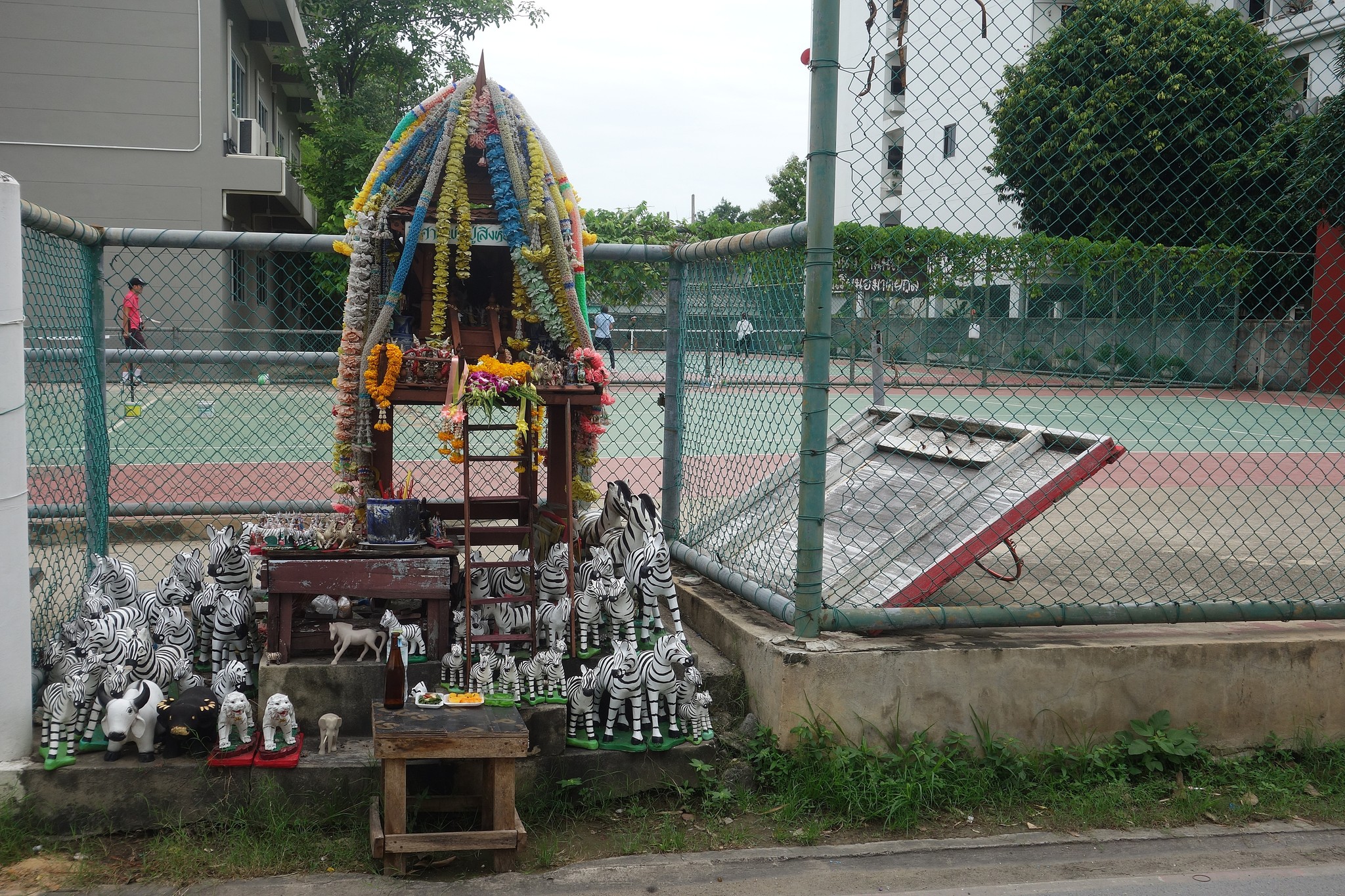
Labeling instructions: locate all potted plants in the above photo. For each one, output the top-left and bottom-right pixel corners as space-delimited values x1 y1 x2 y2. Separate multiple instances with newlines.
927 346 947 361
957 343 980 364
1092 343 1137 373
778 334 793 352
1012 347 1042 367
1052 347 1080 370
1145 355 1192 381
860 341 872 356
837 336 851 355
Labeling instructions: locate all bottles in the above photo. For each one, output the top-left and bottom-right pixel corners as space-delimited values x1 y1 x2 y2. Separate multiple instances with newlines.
386 627 408 702
383 632 405 710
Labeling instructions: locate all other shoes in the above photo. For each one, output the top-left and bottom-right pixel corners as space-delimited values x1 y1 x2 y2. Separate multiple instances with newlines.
738 358 745 364
620 348 625 352
744 359 748 365
633 350 639 354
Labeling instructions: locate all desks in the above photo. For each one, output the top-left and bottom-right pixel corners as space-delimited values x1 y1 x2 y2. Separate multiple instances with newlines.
259 512 458 661
370 694 529 878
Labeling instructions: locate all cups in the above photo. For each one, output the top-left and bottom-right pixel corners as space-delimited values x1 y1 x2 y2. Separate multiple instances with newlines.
366 499 420 544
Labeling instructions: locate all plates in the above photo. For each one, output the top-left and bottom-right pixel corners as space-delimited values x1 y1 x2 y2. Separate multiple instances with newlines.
358 541 428 550
444 694 484 707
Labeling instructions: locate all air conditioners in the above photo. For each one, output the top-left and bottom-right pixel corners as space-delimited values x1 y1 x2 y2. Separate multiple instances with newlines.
237 118 275 157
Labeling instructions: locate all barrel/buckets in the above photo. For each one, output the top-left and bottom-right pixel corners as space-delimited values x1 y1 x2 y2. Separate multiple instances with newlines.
197 401 215 418
258 374 269 385
123 401 142 418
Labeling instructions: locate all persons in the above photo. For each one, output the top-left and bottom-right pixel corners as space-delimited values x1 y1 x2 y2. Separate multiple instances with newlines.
120 278 149 386
590 304 618 373
735 313 754 365
620 316 638 353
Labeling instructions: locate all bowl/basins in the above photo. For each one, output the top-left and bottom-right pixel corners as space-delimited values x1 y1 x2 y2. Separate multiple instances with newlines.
483 689 518 707
415 692 444 708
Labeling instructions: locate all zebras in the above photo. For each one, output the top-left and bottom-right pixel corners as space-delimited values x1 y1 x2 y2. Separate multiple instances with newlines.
253 519 358 551
378 608 427 665
36 519 281 762
438 477 715 746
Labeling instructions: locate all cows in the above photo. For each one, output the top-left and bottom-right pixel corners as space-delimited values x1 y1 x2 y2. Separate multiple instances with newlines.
156 684 220 760
96 678 166 762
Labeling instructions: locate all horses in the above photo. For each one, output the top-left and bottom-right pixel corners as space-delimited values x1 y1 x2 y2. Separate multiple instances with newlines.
327 620 388 666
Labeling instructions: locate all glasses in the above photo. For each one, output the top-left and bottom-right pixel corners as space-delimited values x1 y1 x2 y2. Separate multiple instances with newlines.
138 285 142 287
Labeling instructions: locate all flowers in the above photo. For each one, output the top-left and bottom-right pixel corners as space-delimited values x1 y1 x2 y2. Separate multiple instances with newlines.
331 79 617 522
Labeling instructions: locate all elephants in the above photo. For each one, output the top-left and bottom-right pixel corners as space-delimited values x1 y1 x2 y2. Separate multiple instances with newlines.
317 712 343 756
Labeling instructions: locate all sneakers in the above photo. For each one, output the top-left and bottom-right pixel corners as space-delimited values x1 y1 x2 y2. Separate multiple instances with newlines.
119 375 147 386
611 368 618 373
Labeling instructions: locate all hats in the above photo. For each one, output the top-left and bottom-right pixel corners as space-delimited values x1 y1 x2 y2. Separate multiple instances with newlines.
128 278 147 286
630 316 637 319
